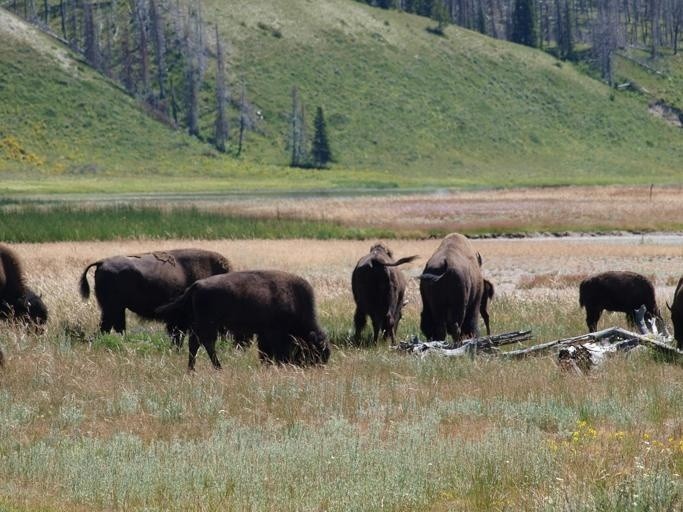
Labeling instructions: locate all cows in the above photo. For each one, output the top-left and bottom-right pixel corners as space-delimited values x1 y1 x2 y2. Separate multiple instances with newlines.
579 271 668 339
414 233 484 343
78 249 254 352
352 244 422 348
666 278 683 351
156 270 329 375
0 281 49 335
420 279 493 342
